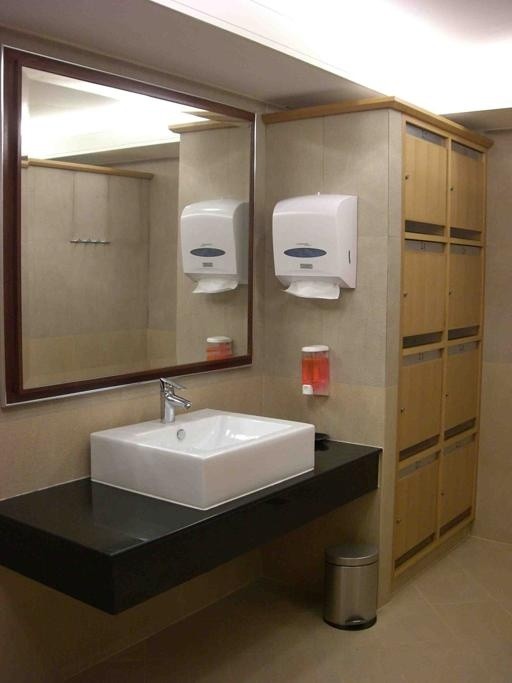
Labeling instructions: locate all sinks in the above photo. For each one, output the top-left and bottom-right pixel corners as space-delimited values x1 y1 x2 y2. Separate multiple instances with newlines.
89 409 316 510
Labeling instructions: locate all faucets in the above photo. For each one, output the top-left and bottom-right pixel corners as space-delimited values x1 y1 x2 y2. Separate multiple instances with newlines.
160 376 191 422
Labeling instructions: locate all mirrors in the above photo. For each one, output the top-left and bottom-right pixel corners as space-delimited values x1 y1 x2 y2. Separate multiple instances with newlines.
1 27 266 408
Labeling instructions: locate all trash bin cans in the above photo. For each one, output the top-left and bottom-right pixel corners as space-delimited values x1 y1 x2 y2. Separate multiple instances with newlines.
322 544 379 631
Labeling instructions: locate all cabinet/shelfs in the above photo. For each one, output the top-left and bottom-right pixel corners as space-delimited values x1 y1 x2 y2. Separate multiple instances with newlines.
265 95 493 608
